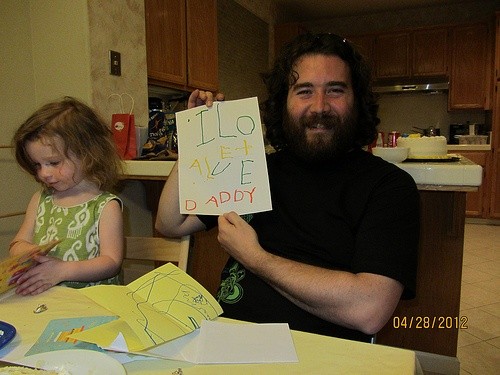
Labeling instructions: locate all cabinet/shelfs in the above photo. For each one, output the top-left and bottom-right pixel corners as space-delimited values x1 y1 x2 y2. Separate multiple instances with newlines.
341 34 374 77
448 19 492 110
144 0 219 96
448 149 489 224
275 23 307 58
493 13 500 226
374 24 449 85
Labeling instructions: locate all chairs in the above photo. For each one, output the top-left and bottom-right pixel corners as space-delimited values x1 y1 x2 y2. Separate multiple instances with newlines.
115 157 190 272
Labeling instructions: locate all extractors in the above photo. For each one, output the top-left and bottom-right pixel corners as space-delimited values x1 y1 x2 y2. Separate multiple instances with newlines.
368 73 452 94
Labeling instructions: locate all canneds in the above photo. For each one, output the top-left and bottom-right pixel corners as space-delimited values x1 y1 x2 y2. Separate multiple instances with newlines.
387 131 401 147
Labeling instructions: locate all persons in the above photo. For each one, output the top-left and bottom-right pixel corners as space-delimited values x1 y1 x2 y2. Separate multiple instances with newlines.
155 33 421 344
7 96 125 295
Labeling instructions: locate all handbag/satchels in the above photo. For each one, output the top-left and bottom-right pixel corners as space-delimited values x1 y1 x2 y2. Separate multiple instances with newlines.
107 92 138 160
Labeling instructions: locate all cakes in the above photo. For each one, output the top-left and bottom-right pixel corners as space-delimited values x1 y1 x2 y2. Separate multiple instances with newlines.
397 133 447 159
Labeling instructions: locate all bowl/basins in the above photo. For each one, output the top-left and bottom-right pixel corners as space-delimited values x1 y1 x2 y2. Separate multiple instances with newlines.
412 125 440 137
371 145 409 164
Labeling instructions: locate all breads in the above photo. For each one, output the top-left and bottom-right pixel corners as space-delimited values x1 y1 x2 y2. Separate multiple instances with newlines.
0 365 58 375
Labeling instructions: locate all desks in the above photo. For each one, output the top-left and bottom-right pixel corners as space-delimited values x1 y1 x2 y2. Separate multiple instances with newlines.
0 282 424 375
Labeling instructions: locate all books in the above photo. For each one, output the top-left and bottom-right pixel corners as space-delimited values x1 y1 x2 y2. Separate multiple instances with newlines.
0 239 64 295
